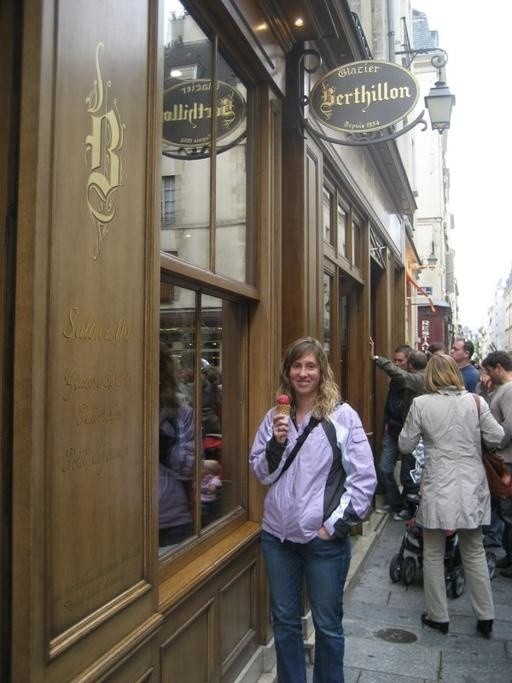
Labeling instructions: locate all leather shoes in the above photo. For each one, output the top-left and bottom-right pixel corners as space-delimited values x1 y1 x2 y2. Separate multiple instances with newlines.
477 620 493 636
495 555 510 567
421 614 448 633
501 566 512 576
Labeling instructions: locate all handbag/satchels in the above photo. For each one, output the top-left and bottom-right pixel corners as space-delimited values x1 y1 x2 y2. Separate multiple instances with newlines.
473 394 511 500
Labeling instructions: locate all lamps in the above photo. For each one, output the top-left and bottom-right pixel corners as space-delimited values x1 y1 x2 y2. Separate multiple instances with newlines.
394 43 457 137
413 236 442 274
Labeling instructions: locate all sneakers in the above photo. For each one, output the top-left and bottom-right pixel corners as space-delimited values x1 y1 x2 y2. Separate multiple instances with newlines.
393 504 419 521
375 502 399 514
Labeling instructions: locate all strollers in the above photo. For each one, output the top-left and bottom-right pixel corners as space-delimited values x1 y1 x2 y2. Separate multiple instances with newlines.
200 433 224 526
388 492 497 601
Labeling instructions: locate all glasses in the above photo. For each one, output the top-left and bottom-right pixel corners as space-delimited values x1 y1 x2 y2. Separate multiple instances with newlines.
464 338 468 343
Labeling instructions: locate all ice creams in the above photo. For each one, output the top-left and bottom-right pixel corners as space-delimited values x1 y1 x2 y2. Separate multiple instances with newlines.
276 394 291 415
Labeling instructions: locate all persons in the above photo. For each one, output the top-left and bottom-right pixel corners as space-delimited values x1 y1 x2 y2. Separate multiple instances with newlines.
159 342 225 552
368 332 512 577
247 335 379 683
396 352 505 637
